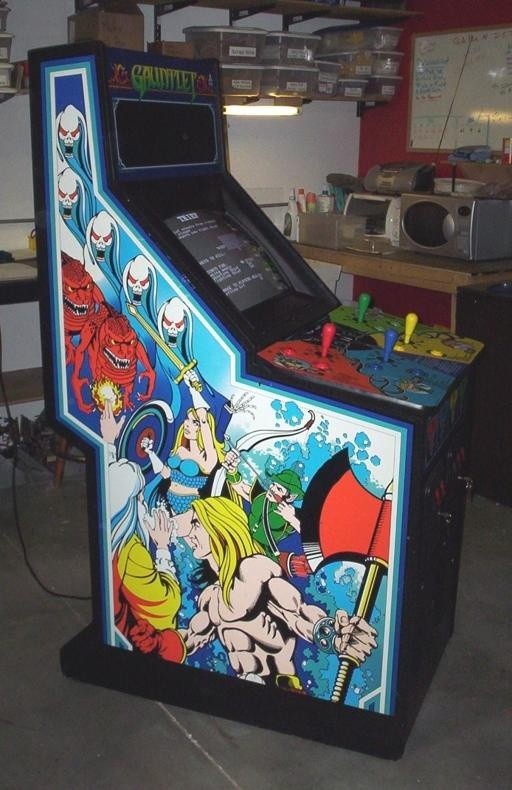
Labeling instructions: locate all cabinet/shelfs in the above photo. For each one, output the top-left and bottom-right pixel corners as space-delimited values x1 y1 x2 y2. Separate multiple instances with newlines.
0 0 423 115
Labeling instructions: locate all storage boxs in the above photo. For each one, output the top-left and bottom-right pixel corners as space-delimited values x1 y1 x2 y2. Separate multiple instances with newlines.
66 3 404 103
299 212 368 251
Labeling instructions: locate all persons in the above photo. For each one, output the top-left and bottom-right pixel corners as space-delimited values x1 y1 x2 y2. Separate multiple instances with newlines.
217 443 304 555
104 456 191 667
174 490 380 694
141 405 228 515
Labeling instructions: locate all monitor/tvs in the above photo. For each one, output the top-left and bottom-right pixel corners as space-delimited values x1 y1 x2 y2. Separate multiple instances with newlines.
151 194 294 314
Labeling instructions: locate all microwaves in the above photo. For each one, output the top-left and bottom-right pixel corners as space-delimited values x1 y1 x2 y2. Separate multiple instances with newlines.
399 192 512 264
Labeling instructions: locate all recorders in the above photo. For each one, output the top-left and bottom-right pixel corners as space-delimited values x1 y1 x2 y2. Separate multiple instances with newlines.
363 161 434 195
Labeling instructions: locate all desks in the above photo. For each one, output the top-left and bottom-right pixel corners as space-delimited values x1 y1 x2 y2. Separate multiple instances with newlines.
292 240 512 336
